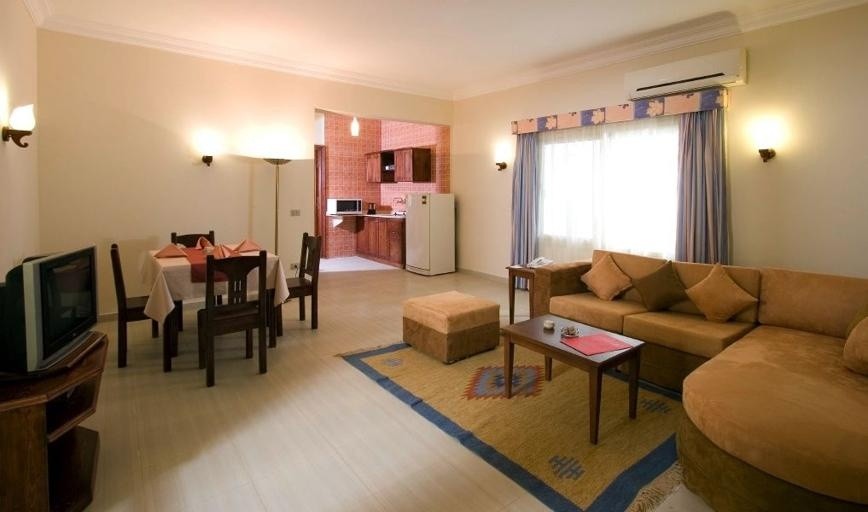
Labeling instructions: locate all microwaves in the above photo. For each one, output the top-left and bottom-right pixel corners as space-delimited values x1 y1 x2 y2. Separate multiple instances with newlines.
327 198 362 215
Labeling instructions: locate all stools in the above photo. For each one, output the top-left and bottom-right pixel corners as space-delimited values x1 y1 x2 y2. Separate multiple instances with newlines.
401 289 500 366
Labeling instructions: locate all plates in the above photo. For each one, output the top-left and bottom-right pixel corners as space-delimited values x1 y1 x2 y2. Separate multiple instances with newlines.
561 327 580 337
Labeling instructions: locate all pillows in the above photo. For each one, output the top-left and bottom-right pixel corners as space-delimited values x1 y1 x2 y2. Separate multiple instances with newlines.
628 256 688 314
579 253 631 302
685 258 761 324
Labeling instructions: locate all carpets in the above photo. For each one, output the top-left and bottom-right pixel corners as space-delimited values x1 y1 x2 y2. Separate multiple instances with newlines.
335 334 684 511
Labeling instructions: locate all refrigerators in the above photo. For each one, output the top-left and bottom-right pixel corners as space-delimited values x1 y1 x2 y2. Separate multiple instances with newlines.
405 191 457 277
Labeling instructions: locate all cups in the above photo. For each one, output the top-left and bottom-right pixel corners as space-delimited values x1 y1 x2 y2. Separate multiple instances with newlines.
542 319 555 330
204 246 214 255
383 165 394 171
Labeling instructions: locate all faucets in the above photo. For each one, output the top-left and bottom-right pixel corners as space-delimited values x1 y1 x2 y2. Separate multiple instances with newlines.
392 197 405 204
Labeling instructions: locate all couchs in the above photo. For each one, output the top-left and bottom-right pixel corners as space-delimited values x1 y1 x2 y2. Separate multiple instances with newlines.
524 250 867 511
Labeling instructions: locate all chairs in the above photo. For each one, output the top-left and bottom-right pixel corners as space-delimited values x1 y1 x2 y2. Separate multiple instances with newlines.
108 229 322 387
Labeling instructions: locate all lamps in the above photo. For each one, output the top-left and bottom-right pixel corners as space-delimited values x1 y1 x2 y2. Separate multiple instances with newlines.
202 153 214 169
494 160 508 170
265 157 291 259
758 148 777 164
1 98 39 152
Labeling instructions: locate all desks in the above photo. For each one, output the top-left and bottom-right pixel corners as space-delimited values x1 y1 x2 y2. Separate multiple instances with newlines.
505 263 538 324
498 314 645 446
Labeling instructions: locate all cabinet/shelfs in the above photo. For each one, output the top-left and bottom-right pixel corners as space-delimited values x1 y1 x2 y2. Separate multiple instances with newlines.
377 215 391 265
354 215 369 260
364 148 396 185
367 215 379 262
392 145 432 184
388 215 406 270
0 332 108 512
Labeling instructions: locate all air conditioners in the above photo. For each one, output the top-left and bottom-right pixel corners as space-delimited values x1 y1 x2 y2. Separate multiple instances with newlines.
619 44 750 104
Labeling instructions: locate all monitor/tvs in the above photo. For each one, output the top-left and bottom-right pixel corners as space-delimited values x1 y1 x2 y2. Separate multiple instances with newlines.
0 244 107 382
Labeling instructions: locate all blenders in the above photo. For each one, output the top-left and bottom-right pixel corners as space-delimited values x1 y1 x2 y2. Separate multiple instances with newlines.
367 202 376 214
